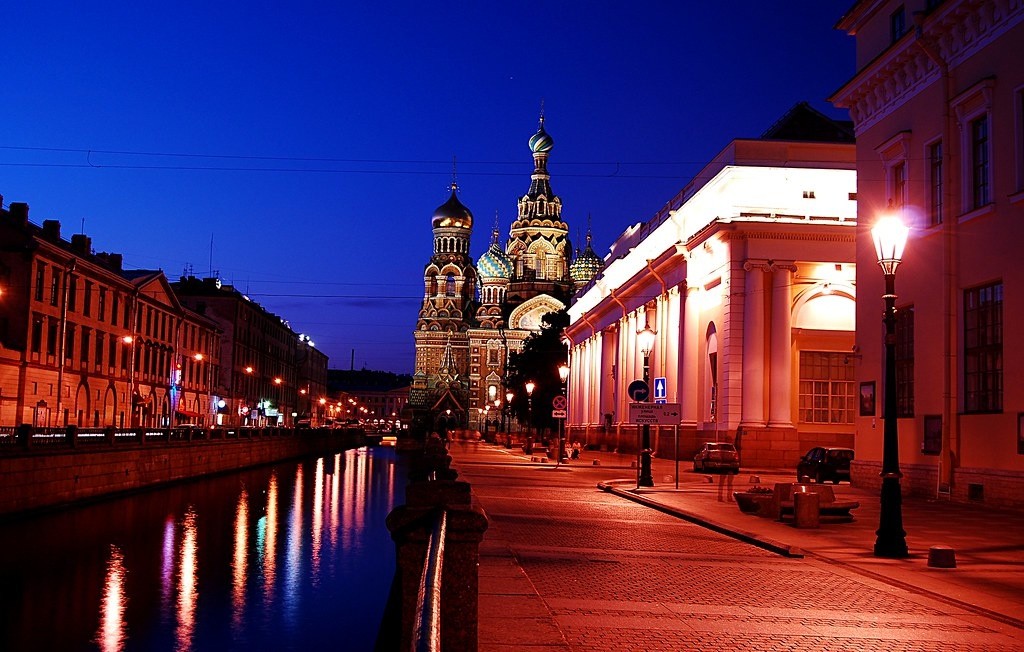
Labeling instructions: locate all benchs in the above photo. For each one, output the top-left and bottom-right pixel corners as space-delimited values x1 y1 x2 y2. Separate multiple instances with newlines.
757 482 859 523
522 443 548 455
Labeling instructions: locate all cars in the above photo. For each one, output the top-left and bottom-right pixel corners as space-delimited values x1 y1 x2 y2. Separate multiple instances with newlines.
691 441 740 473
172 422 281 441
796 446 855 485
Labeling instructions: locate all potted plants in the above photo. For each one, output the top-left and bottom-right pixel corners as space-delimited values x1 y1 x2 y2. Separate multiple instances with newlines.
733 483 774 513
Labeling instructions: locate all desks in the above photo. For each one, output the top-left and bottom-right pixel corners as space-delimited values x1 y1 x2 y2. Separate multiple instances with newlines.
795 492 819 528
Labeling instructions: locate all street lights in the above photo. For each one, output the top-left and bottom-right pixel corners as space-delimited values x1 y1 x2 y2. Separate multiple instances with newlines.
525 379 535 455
485 404 491 434
494 398 501 446
478 408 482 433
556 362 569 461
869 197 913 559
505 389 515 449
635 316 659 487
